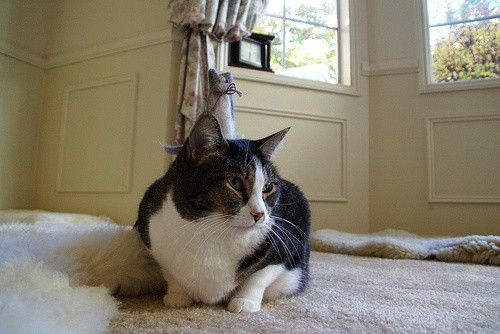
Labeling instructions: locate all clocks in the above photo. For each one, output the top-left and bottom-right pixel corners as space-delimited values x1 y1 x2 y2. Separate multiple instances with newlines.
232 31 275 73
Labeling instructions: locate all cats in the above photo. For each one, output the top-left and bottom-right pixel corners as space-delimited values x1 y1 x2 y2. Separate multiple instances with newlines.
132 110 311 314
